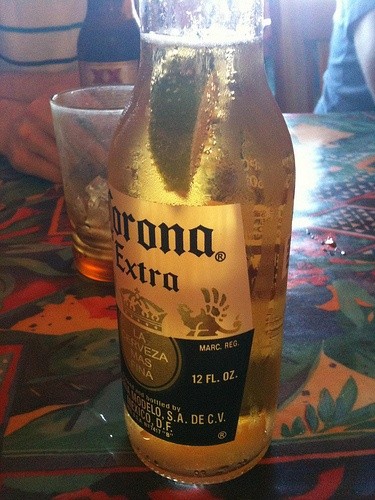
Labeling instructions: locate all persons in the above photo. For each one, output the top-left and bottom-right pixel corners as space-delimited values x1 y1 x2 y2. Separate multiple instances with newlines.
0 0 88 182
313 0 375 114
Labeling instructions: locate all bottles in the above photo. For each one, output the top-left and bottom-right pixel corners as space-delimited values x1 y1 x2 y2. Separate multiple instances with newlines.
109 0 294 483
77 0 142 90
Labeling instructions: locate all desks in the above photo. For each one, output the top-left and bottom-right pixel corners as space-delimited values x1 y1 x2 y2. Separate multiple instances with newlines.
0 111 375 500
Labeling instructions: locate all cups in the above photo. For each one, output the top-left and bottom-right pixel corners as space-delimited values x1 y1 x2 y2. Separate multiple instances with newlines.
52 84 135 282
